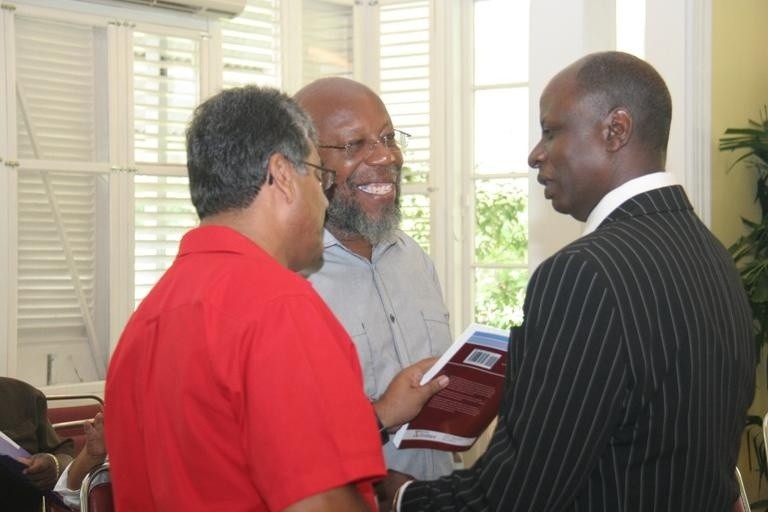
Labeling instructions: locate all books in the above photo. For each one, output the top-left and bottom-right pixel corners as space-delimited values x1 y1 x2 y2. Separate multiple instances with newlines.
393 323 511 454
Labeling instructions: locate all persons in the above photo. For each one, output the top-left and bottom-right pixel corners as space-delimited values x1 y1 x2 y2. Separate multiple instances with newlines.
375 50 756 512
52 411 111 511
101 82 389 512
0 375 76 512
293 77 465 480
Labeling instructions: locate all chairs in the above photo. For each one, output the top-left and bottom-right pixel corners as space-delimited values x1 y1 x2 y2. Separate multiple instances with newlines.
40 395 106 511
78 461 114 512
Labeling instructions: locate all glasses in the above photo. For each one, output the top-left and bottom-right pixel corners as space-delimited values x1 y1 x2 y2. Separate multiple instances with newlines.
267 150 336 191
320 125 413 159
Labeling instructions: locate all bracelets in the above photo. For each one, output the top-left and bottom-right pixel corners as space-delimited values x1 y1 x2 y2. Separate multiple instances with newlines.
48 453 59 481
392 488 400 511
374 413 390 446
